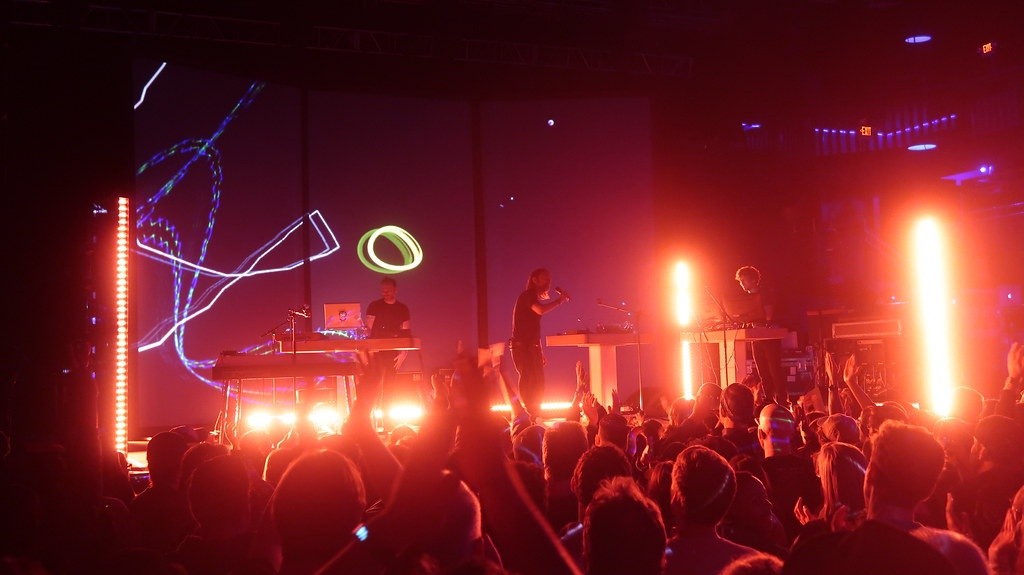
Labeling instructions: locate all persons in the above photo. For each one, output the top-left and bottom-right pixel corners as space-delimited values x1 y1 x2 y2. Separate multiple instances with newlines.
510 267 570 420
0 351 1024 575
730 265 794 401
661 445 764 575
352 276 412 432
781 419 992 575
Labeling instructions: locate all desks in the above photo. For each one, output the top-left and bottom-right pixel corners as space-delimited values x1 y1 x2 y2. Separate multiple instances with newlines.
280 338 422 386
546 334 657 414
680 329 788 390
212 363 357 448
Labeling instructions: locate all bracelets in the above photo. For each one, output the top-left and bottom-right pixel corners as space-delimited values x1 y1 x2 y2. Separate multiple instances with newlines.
574 391 582 397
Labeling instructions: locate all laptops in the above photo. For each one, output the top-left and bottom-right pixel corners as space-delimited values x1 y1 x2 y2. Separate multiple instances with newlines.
324 303 362 329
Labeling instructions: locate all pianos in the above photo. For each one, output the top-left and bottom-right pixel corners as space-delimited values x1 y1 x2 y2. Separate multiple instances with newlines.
279 337 421 353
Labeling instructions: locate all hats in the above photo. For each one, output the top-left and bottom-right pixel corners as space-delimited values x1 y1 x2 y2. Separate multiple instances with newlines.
670 444 737 531
719 382 756 422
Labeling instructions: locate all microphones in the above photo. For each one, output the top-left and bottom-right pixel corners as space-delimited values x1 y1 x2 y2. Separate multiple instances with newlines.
287 304 311 321
556 286 570 302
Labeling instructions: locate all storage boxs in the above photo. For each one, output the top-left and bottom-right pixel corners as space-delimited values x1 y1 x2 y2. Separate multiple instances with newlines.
832 318 900 338
